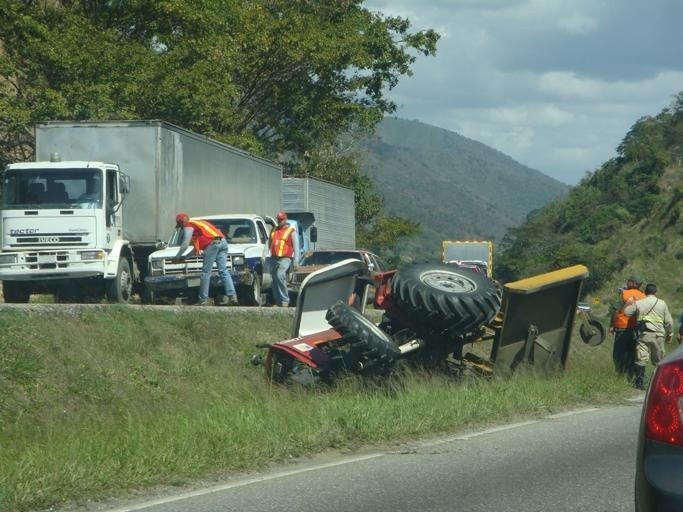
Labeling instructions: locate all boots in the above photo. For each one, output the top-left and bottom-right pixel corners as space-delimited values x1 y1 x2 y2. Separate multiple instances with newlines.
634 365 646 391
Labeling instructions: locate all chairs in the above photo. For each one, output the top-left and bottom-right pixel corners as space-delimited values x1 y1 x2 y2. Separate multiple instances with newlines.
23 182 69 203
234 226 251 238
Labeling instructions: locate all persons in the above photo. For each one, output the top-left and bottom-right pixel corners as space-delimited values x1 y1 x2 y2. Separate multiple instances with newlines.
606 275 647 380
172 211 240 305
621 283 673 392
259 208 300 307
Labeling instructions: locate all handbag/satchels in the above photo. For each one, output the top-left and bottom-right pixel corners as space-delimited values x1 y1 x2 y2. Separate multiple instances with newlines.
631 321 646 341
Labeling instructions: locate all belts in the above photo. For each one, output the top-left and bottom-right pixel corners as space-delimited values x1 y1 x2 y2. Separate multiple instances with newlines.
214 236 223 241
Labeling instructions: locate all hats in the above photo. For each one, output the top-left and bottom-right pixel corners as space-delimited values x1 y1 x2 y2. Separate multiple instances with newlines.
646 283 658 293
175 212 186 228
624 275 644 286
276 212 287 221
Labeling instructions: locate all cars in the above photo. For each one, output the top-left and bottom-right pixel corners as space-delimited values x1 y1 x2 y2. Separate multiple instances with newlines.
289 249 390 304
575 301 591 311
145 214 277 305
635 339 682 510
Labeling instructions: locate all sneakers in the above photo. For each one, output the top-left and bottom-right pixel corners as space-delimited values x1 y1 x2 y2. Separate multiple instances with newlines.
272 302 289 308
194 299 211 306
228 294 240 306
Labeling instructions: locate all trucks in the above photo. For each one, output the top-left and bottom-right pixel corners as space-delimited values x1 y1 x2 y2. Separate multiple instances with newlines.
286 174 356 260
1 117 285 303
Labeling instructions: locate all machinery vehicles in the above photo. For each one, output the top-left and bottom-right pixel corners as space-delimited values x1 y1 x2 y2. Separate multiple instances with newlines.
248 256 608 402
